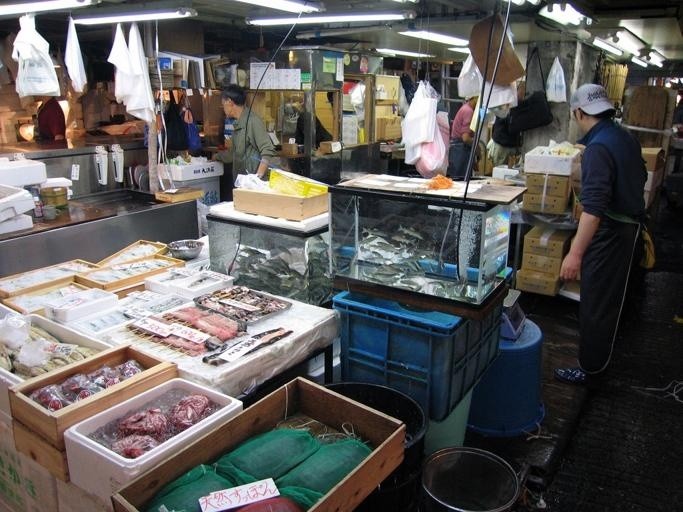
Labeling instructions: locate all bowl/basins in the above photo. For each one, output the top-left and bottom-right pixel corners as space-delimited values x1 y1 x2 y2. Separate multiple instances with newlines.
166 240 204 258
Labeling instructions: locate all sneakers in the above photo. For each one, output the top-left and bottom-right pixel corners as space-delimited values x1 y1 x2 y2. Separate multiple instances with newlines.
555 368 600 390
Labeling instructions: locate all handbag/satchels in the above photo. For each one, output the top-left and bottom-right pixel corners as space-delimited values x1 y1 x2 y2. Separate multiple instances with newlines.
509 91 553 131
492 115 522 146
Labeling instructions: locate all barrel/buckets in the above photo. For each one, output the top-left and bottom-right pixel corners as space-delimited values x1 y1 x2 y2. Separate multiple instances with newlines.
467 319 545 437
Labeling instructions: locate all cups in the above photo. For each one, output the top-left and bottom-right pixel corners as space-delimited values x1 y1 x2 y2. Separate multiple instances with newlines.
41 205 60 221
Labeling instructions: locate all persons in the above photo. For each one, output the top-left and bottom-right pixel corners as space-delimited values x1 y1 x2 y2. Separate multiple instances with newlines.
212 85 276 183
449 91 481 178
33 77 66 142
556 82 650 394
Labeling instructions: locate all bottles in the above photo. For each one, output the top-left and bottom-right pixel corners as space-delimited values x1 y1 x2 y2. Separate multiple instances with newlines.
32 193 43 218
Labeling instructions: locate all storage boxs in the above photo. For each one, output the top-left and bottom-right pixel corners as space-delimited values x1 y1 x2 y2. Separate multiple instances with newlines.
513 143 666 296
373 73 401 142
279 44 384 90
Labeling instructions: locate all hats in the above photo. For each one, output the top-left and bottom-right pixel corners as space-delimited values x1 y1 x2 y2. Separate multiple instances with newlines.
570 84 616 118
